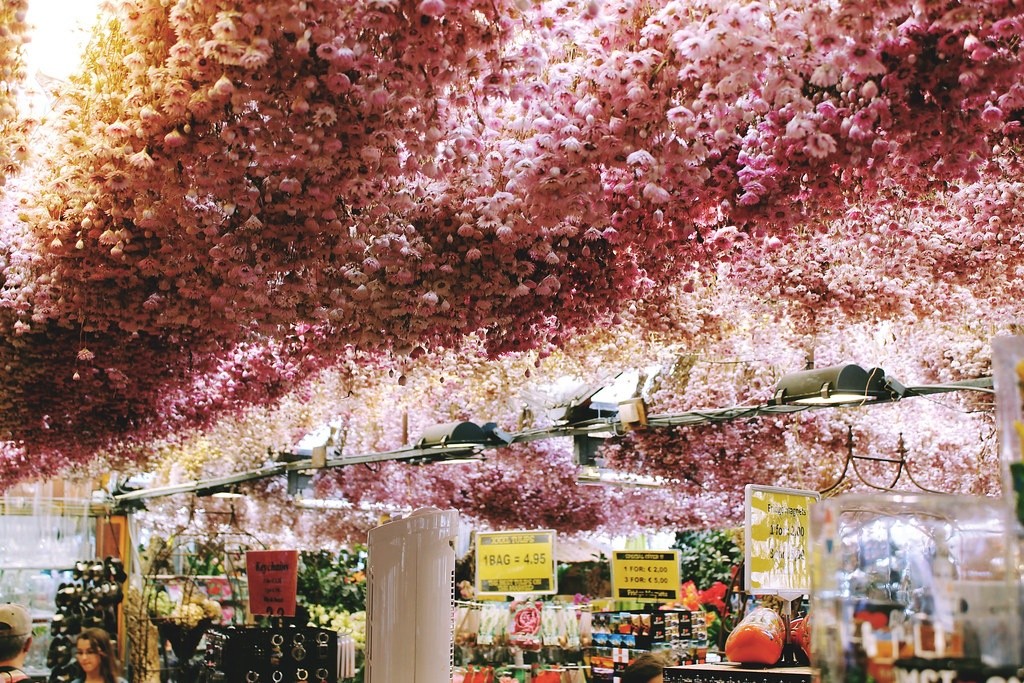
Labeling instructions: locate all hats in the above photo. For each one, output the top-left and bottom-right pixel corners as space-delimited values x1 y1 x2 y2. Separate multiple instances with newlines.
0 601 38 637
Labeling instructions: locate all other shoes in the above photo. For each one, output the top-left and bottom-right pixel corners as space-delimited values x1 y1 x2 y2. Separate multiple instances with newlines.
46 555 128 683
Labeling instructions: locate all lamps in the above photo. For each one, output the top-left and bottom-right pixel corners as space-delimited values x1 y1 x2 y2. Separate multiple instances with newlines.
618 399 650 432
194 483 247 497
775 363 880 405
418 421 487 449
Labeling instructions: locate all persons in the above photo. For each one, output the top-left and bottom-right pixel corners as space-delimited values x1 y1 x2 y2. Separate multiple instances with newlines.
561 564 590 596
620 655 663 683
0 601 40 683
71 627 128 683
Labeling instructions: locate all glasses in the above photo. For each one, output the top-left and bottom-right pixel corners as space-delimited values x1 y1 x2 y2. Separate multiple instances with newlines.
73 648 101 658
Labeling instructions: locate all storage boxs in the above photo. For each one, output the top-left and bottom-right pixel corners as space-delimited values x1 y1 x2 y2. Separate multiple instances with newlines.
932 579 1024 666
